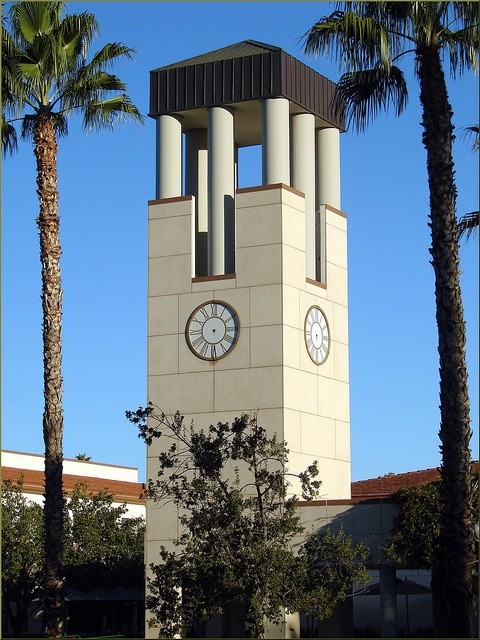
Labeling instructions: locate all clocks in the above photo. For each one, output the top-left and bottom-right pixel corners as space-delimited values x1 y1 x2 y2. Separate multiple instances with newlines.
184 299 241 361
303 305 330 366
